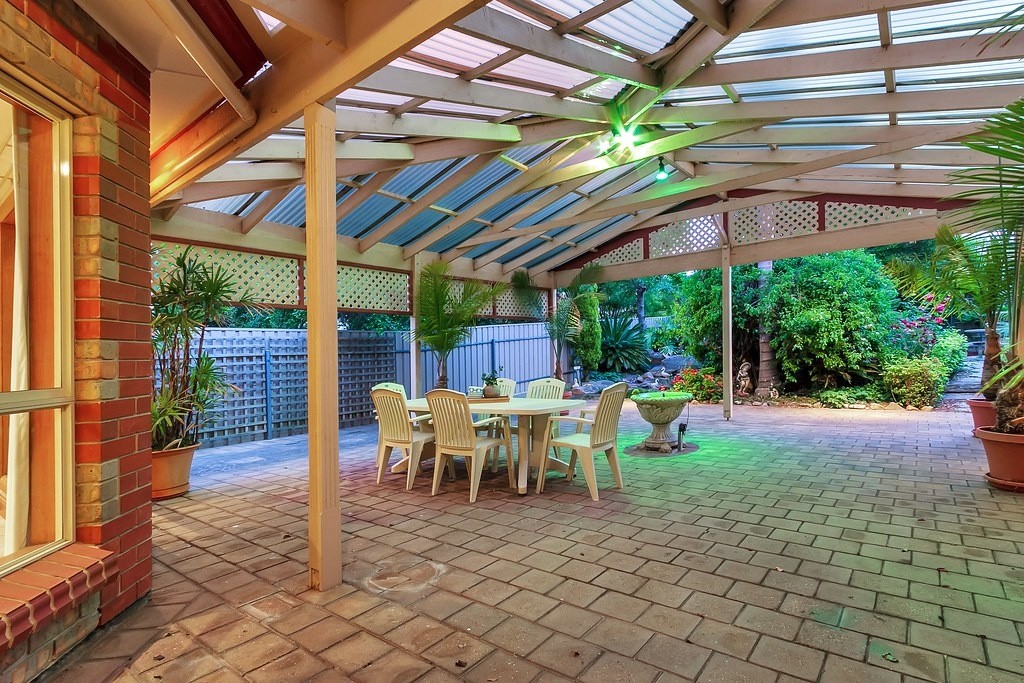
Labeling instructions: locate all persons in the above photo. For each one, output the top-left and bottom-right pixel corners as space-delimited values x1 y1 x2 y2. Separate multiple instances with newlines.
736 361 751 396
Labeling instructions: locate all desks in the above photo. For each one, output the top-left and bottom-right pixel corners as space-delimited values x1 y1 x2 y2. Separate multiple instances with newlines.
406 396 587 497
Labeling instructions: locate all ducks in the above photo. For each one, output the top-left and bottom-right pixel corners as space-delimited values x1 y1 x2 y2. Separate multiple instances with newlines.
650 379 659 388
661 367 672 378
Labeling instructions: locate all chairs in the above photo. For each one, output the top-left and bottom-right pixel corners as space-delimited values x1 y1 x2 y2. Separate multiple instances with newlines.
371 382 429 475
424 389 517 503
535 381 630 502
369 387 456 491
492 378 566 479
474 377 516 472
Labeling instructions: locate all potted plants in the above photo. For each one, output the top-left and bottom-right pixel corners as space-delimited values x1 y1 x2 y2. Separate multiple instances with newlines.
510 260 604 416
482 365 503 397
151 240 277 502
935 95 1024 493
399 260 515 391
885 222 1013 438
